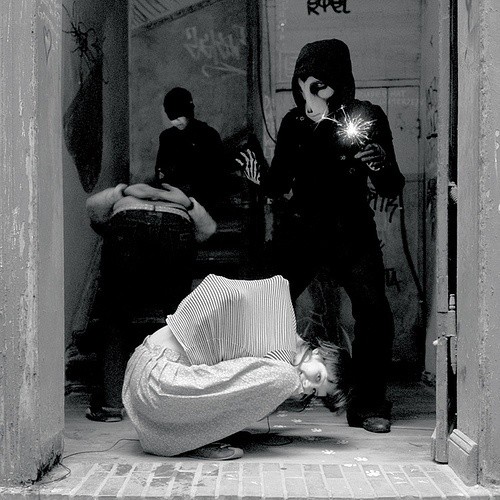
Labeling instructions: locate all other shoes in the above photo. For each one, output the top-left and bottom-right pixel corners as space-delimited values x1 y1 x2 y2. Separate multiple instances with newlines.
85 407 121 422
182 441 244 460
363 416 391 433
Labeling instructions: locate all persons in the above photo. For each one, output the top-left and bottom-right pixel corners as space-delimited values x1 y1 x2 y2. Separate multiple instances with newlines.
233 37 404 433
121 274 340 461
82 184 218 423
152 87 228 220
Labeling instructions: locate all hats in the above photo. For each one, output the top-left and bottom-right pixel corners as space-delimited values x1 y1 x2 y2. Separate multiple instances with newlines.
163 87 195 121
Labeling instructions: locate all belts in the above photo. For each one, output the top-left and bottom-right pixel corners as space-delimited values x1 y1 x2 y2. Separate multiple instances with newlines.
111 204 191 221
